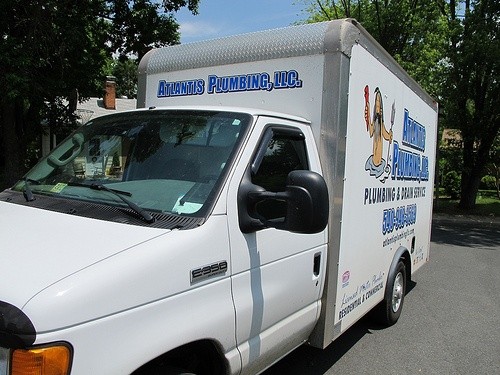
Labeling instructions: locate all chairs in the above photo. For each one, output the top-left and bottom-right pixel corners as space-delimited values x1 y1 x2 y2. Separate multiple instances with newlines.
126 155 203 189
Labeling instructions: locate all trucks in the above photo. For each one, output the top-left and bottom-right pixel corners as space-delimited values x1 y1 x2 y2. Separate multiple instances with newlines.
1 16 438 371
